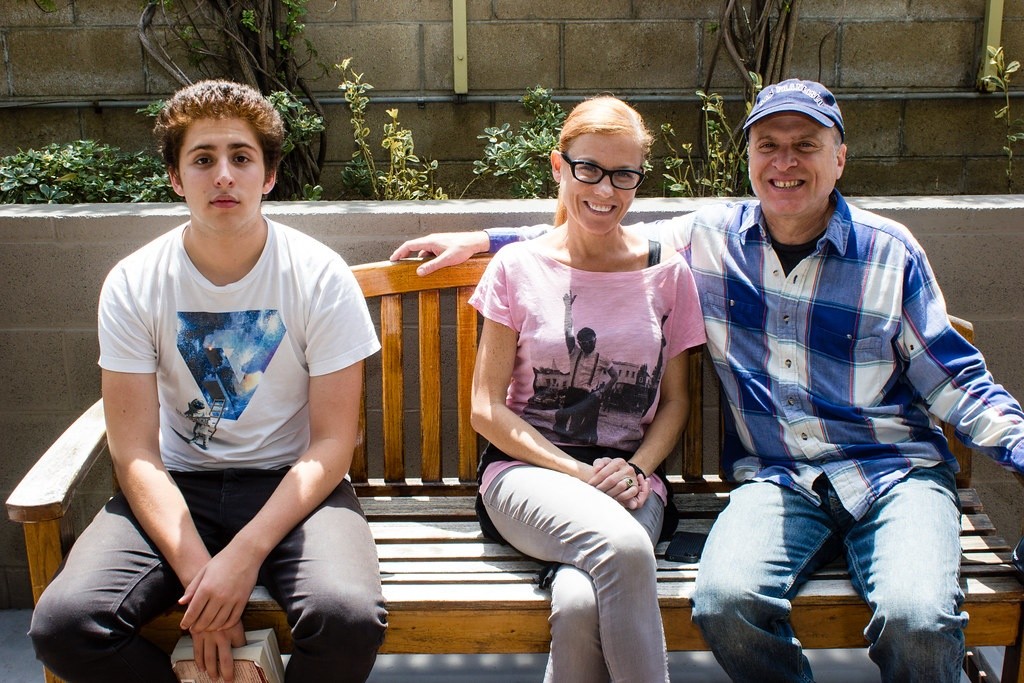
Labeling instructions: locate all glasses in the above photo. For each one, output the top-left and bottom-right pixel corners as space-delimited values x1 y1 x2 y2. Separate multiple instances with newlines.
561 152 646 190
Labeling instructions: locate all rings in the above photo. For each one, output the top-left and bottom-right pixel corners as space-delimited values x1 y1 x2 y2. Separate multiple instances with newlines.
625 479 634 487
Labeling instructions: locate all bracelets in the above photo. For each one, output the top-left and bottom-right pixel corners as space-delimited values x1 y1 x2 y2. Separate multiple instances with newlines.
628 463 647 480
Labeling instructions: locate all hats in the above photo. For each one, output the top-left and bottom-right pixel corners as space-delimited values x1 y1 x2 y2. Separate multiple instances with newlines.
743 79 844 143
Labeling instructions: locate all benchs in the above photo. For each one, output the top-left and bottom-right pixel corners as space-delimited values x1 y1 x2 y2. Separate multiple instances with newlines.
4 254 1024 683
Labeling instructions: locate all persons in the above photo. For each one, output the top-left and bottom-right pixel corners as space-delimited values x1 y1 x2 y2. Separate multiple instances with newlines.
28 78 389 683
467 97 706 683
389 78 1024 683
